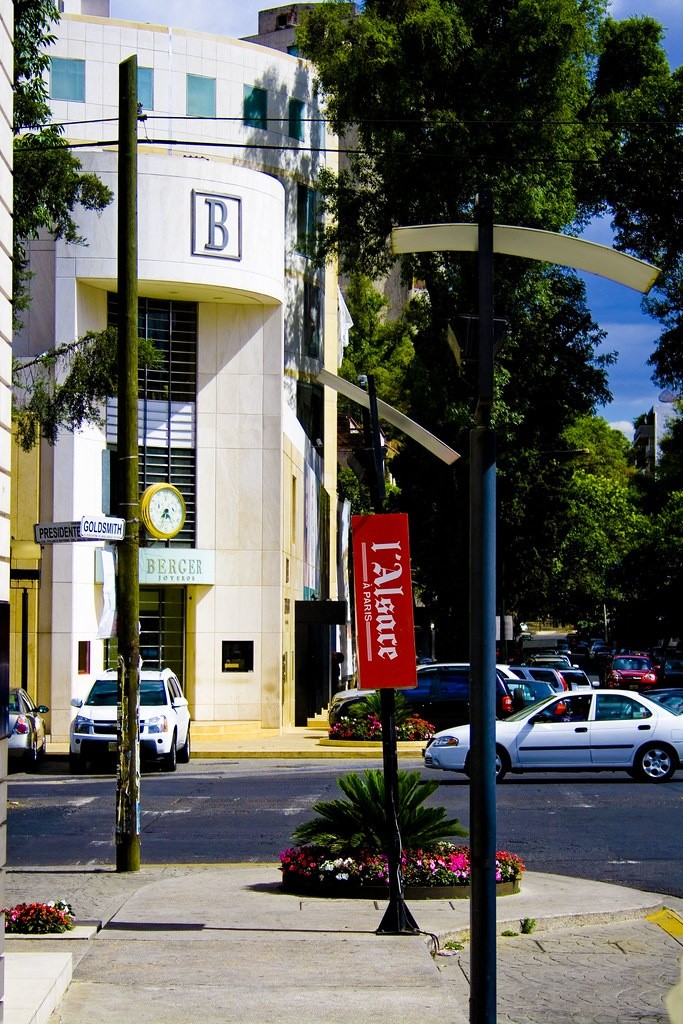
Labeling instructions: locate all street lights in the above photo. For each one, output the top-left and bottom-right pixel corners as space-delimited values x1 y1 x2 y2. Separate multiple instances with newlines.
387 180 662 1024
313 368 463 817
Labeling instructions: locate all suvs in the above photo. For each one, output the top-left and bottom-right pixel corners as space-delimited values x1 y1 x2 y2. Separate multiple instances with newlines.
70 669 192 773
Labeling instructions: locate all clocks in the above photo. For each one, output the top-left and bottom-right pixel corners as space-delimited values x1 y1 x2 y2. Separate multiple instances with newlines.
139 482 186 539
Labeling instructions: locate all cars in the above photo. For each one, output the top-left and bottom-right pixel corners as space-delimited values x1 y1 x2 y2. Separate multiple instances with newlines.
421 686 683 785
7 685 49 773
331 629 683 723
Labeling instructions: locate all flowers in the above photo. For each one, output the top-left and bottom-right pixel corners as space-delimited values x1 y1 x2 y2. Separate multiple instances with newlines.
328 713 434 742
276 847 524 891
0 900 77 934
